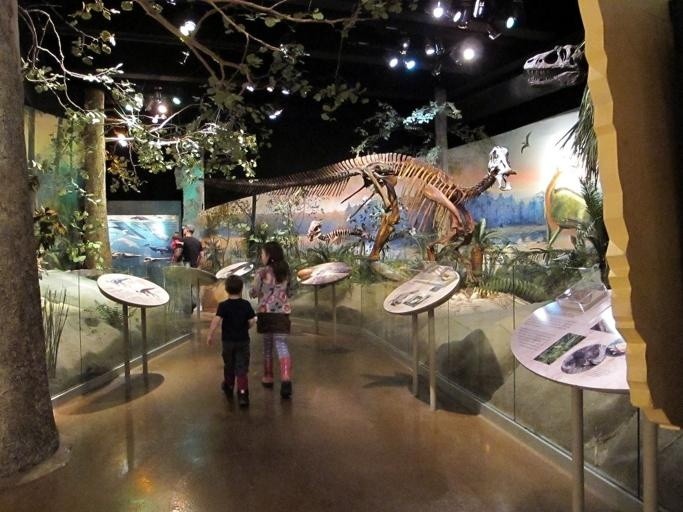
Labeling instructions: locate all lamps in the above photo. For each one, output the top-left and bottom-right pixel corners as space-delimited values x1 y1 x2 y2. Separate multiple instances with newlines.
266 79 276 92
181 15 203 36
245 78 259 93
385 1 516 76
263 100 282 120
282 87 290 96
156 93 184 114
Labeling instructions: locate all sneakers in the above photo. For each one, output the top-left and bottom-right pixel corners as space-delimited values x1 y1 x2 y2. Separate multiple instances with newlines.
221 380 233 399
235 390 250 408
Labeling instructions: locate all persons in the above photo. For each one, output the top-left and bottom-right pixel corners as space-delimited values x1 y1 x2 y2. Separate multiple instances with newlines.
173 225 205 312
206 275 256 406
249 241 292 398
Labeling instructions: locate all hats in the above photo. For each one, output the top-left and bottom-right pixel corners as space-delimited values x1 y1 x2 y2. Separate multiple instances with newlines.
180 224 194 232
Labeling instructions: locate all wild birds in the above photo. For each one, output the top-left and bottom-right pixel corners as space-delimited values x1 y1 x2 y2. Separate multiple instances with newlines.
520 130 532 153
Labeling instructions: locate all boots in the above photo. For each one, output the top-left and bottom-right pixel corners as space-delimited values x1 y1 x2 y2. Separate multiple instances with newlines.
260 356 273 386
278 356 294 397
